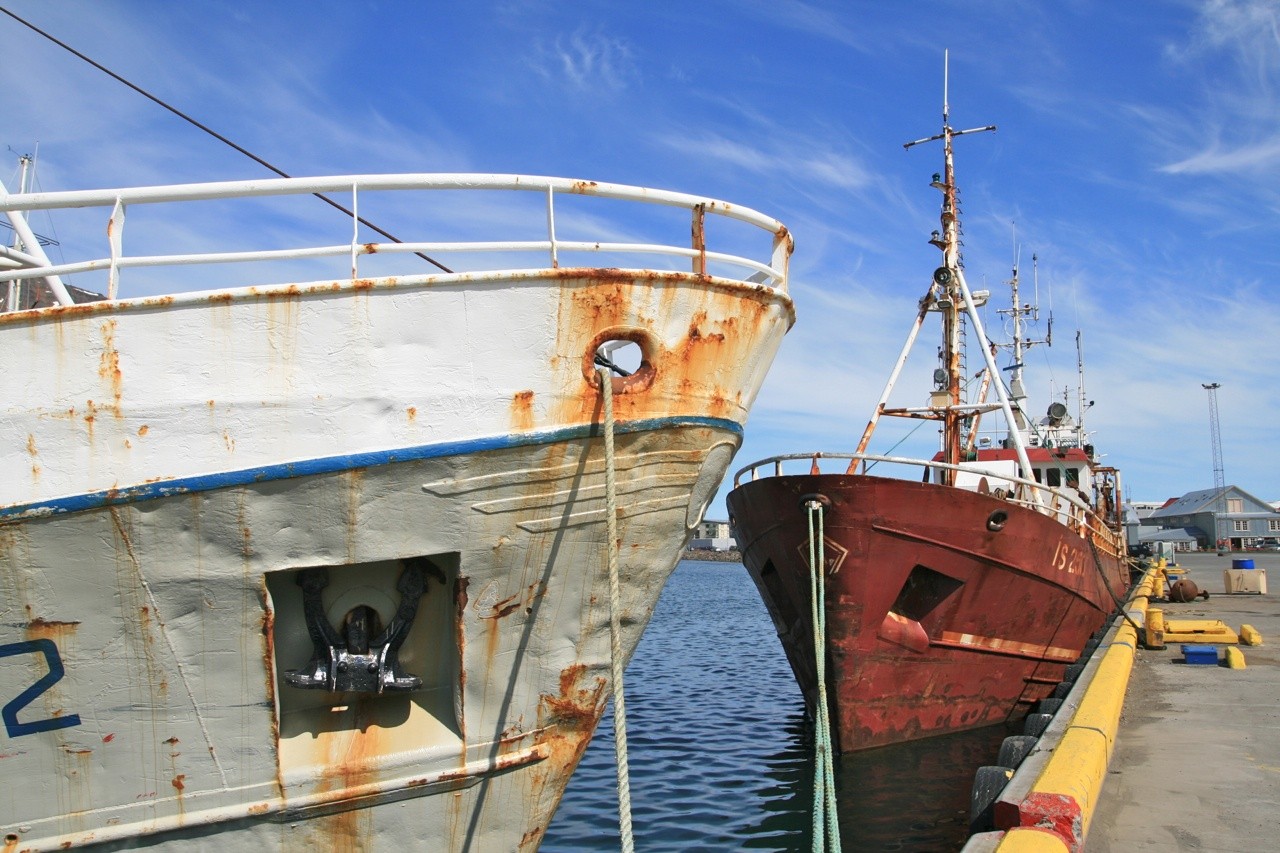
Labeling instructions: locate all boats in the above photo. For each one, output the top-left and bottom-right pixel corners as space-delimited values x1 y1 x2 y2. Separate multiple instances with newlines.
722 46 1134 753
2 177 796 853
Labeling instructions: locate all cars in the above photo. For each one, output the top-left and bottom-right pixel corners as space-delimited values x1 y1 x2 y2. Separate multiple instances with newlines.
1246 539 1278 548
1126 544 1153 559
710 547 721 552
729 546 737 551
